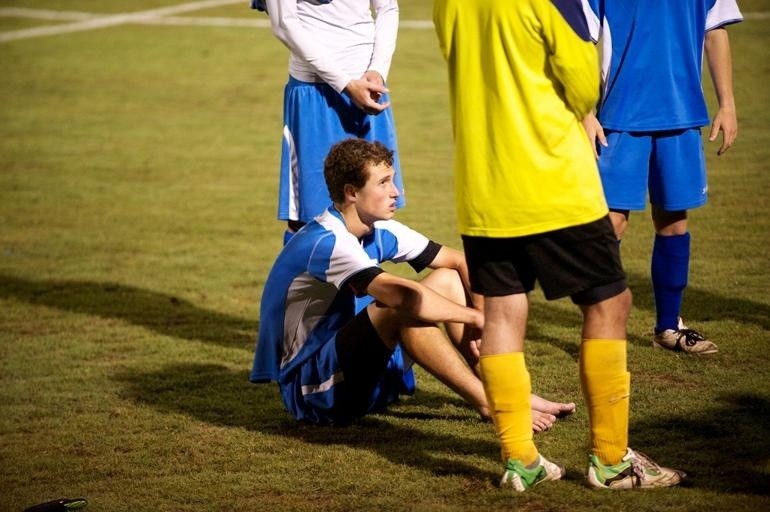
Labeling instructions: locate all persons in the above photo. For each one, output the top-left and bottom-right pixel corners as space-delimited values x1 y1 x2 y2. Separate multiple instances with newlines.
265 0 406 244
583 0 745 356
433 0 696 492
250 139 576 432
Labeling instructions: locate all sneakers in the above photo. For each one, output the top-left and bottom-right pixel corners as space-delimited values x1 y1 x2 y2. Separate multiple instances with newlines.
584 444 686 488
654 315 721 354
501 449 565 493
